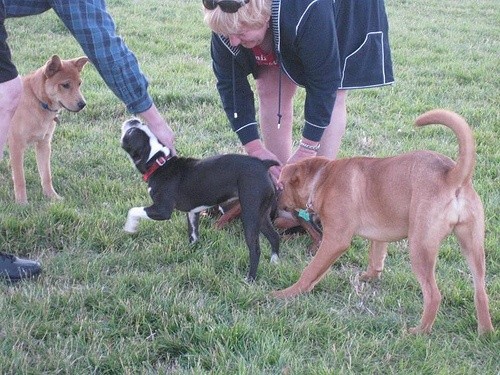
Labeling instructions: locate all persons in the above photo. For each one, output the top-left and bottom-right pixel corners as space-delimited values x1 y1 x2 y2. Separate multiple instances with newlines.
0 0 177 283
201 0 395 236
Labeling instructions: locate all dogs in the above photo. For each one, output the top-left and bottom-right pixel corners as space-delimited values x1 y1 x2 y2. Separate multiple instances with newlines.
7 54 90 205
119 109 495 336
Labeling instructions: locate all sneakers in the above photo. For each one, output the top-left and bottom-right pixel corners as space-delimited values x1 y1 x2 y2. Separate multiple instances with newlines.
0 252 42 282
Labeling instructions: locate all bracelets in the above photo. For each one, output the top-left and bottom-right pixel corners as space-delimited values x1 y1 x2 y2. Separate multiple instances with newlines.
300 139 321 152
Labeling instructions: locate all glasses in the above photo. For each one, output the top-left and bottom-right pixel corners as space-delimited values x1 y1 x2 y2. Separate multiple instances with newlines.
202 0 249 13
